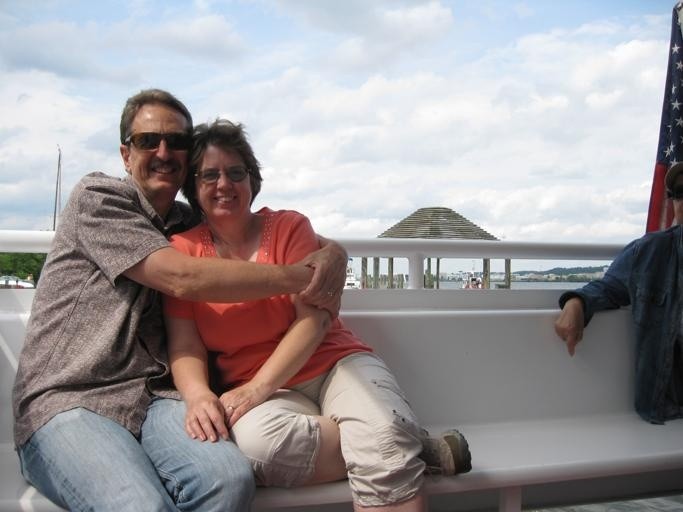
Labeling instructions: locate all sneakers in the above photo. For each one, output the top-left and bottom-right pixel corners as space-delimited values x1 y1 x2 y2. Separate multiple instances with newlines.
420 427 471 483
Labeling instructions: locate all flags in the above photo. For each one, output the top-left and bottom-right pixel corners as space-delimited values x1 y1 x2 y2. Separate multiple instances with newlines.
645 7 681 234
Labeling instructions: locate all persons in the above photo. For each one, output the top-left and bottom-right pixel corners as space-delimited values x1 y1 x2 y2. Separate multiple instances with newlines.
157 118 472 512
553 161 682 425
10 89 349 512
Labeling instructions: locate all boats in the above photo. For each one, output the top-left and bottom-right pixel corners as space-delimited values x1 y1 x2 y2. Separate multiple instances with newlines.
460 277 481 289
343 259 361 291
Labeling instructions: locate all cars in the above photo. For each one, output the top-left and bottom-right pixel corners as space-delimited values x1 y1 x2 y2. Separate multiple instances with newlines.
0 275 34 289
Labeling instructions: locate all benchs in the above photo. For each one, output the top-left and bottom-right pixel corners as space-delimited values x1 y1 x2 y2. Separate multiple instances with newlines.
1 310 683 512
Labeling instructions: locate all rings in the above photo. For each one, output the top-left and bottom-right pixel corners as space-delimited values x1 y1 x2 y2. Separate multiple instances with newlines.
327 287 336 297
227 405 235 412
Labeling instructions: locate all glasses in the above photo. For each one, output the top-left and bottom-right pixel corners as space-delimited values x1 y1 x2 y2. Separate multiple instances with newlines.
193 166 251 184
125 132 191 150
